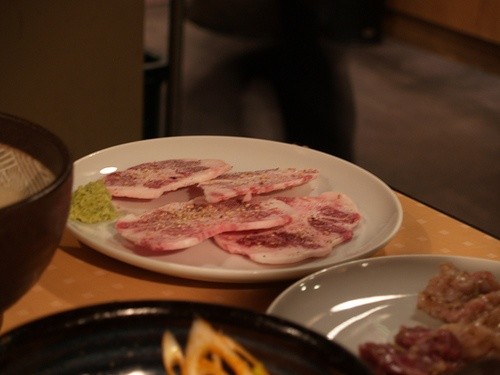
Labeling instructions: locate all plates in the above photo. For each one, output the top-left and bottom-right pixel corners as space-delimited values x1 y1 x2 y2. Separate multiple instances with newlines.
263 254 500 359
62 135 404 283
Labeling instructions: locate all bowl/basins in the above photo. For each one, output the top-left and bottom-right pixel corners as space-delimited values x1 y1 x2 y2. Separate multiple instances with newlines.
0 110 73 313
1 298 364 375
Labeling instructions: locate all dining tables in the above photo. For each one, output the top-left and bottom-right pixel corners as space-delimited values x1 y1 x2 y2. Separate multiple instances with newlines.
0 190 500 334
